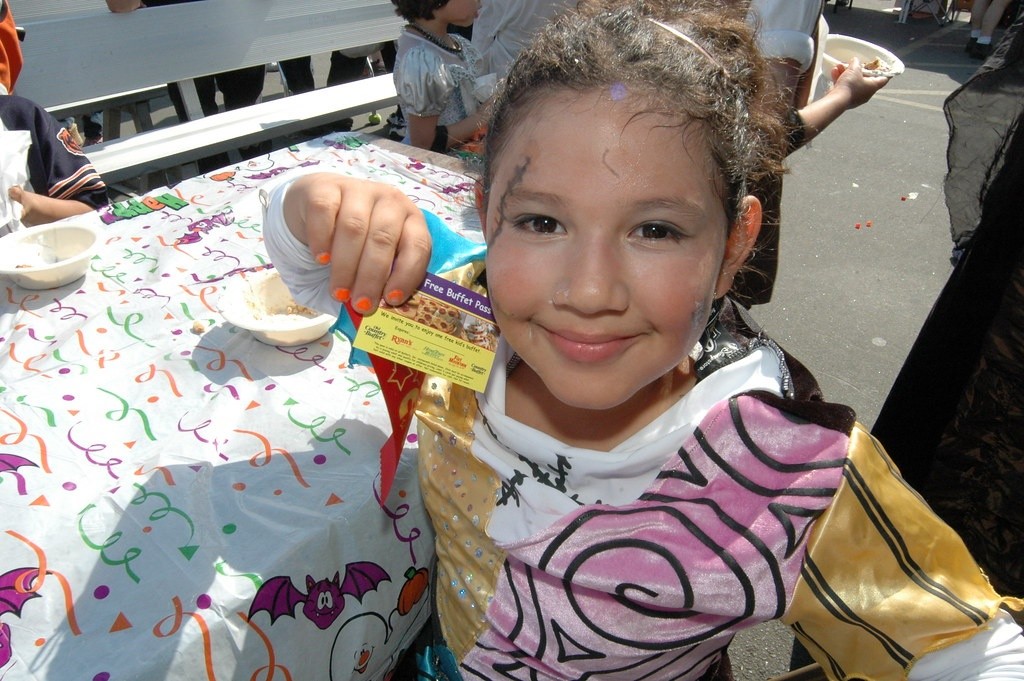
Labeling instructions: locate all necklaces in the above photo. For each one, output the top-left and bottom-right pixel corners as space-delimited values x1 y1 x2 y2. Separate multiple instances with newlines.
405 22 463 54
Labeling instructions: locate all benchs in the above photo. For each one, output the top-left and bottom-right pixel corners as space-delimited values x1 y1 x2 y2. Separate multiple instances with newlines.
43 83 169 190
12 0 409 191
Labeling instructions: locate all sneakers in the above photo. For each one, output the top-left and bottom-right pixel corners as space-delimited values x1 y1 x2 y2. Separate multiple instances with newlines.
967 37 980 51
968 43 993 60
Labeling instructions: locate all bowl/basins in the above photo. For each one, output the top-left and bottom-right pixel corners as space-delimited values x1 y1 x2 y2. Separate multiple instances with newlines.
821 34 905 80
0 221 100 291
219 271 341 347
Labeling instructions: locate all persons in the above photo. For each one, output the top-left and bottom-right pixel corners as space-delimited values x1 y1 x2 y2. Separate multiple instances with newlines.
731 0 889 312
391 0 497 156
104 0 273 175
278 50 367 138
0 94 108 240
58 110 105 149
471 0 580 81
260 0 1024 681
964 0 1012 59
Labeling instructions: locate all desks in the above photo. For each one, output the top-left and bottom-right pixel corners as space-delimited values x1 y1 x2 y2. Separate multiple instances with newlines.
0 128 486 680
